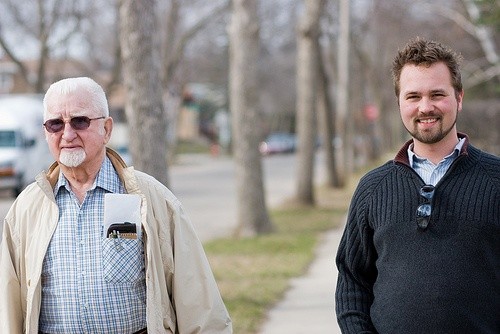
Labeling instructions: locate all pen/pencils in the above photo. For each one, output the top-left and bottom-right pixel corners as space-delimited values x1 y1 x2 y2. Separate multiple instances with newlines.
112 230 117 238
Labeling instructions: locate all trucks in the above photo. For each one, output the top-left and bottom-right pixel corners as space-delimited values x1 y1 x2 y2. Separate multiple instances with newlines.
0 97 54 198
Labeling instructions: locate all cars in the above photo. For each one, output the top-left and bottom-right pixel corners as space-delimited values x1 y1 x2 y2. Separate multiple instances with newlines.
260 131 294 156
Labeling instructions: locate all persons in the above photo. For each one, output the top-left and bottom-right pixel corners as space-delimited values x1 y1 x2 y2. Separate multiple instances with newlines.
335 40 500 334
0 77 234 334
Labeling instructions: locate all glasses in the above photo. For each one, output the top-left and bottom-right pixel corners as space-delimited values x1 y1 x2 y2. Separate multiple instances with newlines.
415 184 435 230
43 116 106 134
